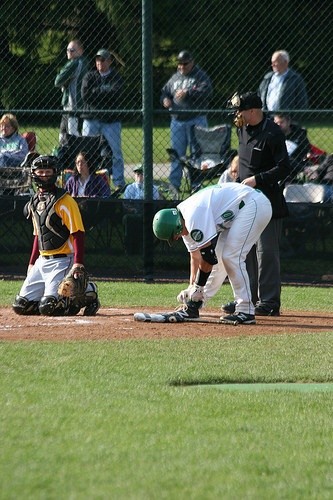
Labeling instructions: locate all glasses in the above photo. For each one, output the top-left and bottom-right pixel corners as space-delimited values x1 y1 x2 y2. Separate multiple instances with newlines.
67 48 76 52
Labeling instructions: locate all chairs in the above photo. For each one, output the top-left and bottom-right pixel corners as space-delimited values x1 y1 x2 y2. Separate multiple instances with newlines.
0 120 333 205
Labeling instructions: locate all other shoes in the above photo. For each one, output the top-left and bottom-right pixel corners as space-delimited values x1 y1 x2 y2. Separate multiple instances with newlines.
115 185 121 191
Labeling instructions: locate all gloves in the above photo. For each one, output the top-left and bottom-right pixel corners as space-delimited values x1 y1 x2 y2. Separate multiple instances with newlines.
177 284 194 303
189 284 203 302
66 264 90 297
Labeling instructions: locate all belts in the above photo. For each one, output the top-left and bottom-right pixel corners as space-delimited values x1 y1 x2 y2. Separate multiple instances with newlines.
239 200 245 209
44 253 67 259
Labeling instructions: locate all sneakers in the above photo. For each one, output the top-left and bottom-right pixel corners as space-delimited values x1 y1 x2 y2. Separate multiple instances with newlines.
221 301 236 314
254 302 280 316
174 304 199 318
220 311 256 324
83 282 100 316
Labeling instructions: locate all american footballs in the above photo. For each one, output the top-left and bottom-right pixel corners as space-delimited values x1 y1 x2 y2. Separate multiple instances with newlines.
57 280 74 296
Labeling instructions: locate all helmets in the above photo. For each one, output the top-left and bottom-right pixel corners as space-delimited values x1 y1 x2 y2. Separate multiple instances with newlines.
31 155 57 188
153 208 183 248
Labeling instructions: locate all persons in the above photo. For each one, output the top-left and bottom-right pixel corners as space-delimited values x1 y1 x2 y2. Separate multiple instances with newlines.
122 164 163 200
160 50 213 194
258 50 307 128
0 113 29 167
153 182 272 324
221 91 290 315
273 112 311 176
54 39 88 148
11 154 101 316
218 155 240 184
309 153 333 203
81 49 127 191
64 151 111 197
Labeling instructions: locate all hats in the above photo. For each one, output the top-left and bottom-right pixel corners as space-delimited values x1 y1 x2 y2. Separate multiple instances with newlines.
95 48 110 59
227 90 263 110
178 50 193 64
133 164 143 175
